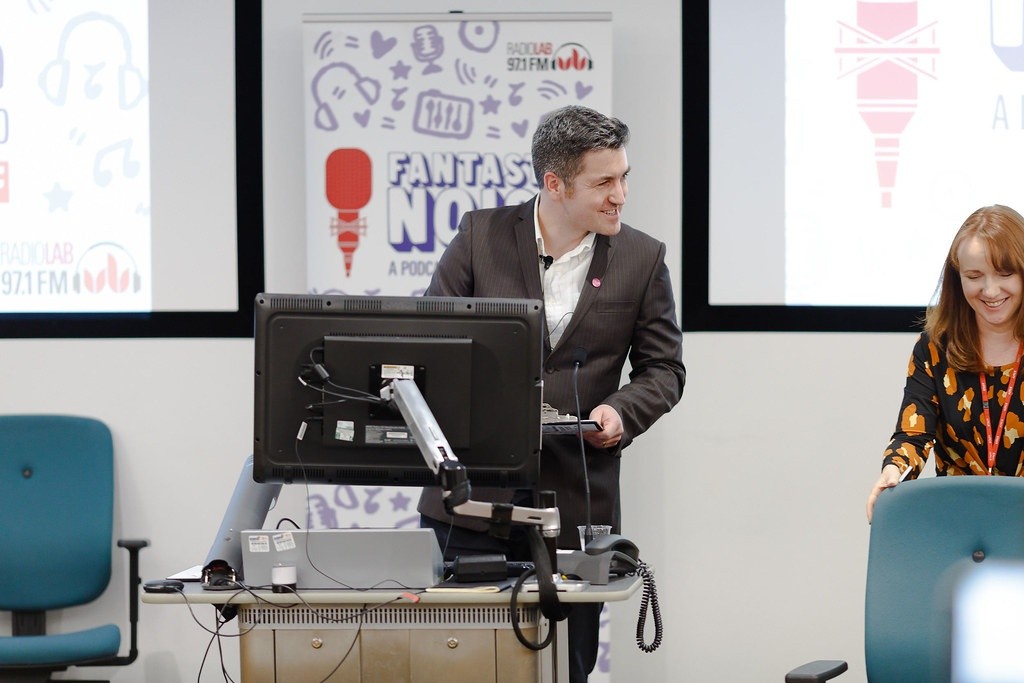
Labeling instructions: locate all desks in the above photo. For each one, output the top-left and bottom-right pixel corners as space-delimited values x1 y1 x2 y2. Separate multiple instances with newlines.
142 566 655 683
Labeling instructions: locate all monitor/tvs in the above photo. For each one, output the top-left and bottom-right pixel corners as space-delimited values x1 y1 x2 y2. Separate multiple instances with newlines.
253 292 543 486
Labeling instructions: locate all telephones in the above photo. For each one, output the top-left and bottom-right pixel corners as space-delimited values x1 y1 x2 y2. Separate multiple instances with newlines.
556 532 640 585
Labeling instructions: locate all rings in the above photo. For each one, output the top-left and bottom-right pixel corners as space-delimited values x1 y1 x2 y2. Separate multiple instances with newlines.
602 441 607 448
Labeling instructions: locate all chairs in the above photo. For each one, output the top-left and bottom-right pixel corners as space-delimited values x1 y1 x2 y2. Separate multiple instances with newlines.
0 414 148 683
785 475 1024 683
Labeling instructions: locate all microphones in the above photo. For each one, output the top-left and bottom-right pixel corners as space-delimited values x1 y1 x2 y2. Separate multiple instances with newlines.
572 346 593 547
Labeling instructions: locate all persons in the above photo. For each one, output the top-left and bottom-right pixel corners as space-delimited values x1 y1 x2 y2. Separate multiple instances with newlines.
421 104 687 683
866 204 1024 525
